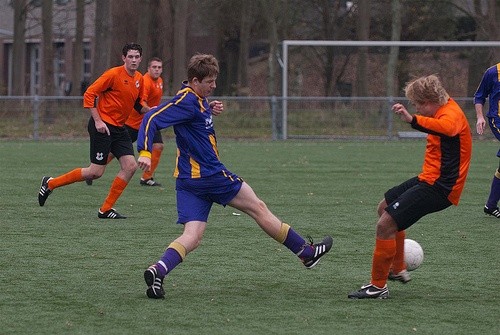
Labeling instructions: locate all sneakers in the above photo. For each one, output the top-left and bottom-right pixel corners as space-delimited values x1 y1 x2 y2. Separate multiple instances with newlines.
140 177 161 186
348 282 389 299
388 268 412 283
300 235 333 268
484 204 500 218
144 263 165 298
98 207 127 219
86 179 92 185
38 176 54 207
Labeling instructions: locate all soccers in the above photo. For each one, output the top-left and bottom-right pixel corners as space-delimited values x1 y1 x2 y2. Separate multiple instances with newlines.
404 238 424 271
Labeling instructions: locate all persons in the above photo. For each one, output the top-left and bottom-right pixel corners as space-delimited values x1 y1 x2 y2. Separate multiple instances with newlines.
37 43 149 218
347 73 472 300
135 55 333 299
85 57 164 186
474 61 500 218
